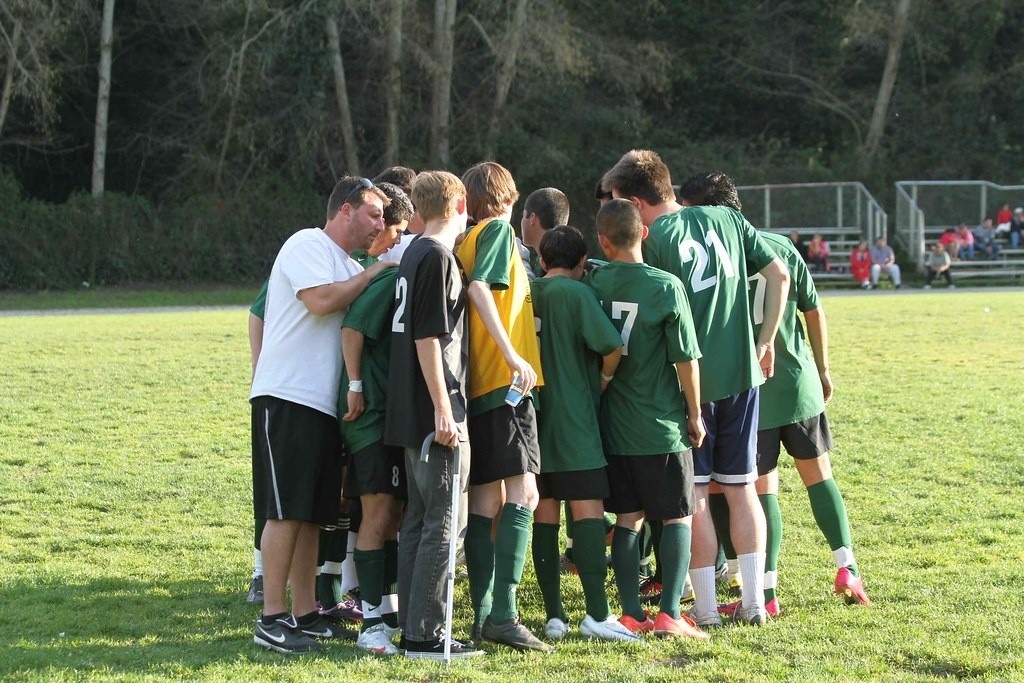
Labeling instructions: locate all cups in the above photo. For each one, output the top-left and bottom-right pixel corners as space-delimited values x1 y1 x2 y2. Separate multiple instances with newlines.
505 375 526 407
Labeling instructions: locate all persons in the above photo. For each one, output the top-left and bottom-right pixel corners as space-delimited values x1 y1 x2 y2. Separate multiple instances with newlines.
973 217 1003 259
997 203 1024 249
790 230 808 264
244 148 872 658
807 233 831 272
870 236 901 290
924 243 955 289
940 222 974 261
850 241 873 289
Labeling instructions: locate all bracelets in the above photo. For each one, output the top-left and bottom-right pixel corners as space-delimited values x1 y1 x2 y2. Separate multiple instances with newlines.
601 372 614 382
348 380 363 392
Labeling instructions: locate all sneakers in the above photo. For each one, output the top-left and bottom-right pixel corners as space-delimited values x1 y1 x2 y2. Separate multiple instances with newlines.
246 574 264 603
470 624 484 644
607 569 654 590
835 568 870 605
314 597 364 625
396 634 486 659
382 622 402 636
614 578 665 598
652 611 712 640
355 623 400 655
340 586 363 612
638 590 696 605
620 610 656 634
683 605 722 629
480 614 555 653
546 617 570 640
729 603 767 627
729 578 743 596
717 598 780 617
298 612 359 643
254 611 320 654
579 615 641 641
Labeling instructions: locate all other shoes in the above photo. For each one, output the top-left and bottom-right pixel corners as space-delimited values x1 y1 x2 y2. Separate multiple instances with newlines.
604 524 616 545
562 555 578 574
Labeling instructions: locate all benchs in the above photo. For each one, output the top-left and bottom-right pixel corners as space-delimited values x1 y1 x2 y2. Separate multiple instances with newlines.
765 229 1024 284
